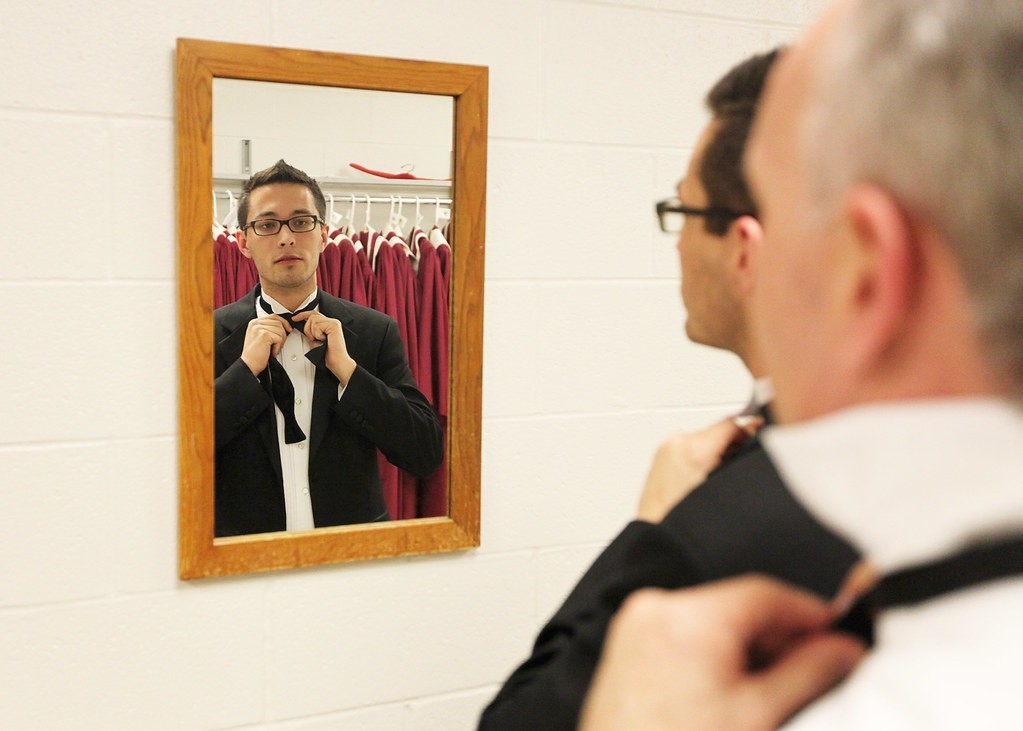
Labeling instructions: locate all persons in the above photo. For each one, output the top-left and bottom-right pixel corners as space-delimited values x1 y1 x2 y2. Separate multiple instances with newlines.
474 48 863 731
581 0 1023 731
213 159 444 539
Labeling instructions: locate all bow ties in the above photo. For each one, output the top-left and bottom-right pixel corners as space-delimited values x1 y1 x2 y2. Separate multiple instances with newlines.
258 293 327 443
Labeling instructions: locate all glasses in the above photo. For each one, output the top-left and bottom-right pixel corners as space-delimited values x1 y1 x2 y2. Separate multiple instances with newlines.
655 194 753 234
243 214 325 236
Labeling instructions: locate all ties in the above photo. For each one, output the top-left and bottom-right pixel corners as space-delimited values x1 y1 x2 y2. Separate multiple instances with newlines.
832 528 1022 645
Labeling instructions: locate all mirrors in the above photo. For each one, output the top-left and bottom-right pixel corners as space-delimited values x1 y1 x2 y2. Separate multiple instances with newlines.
175 36 489 582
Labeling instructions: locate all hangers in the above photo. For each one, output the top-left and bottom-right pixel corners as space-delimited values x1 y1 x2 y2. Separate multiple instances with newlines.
349 161 453 182
211 188 440 230
